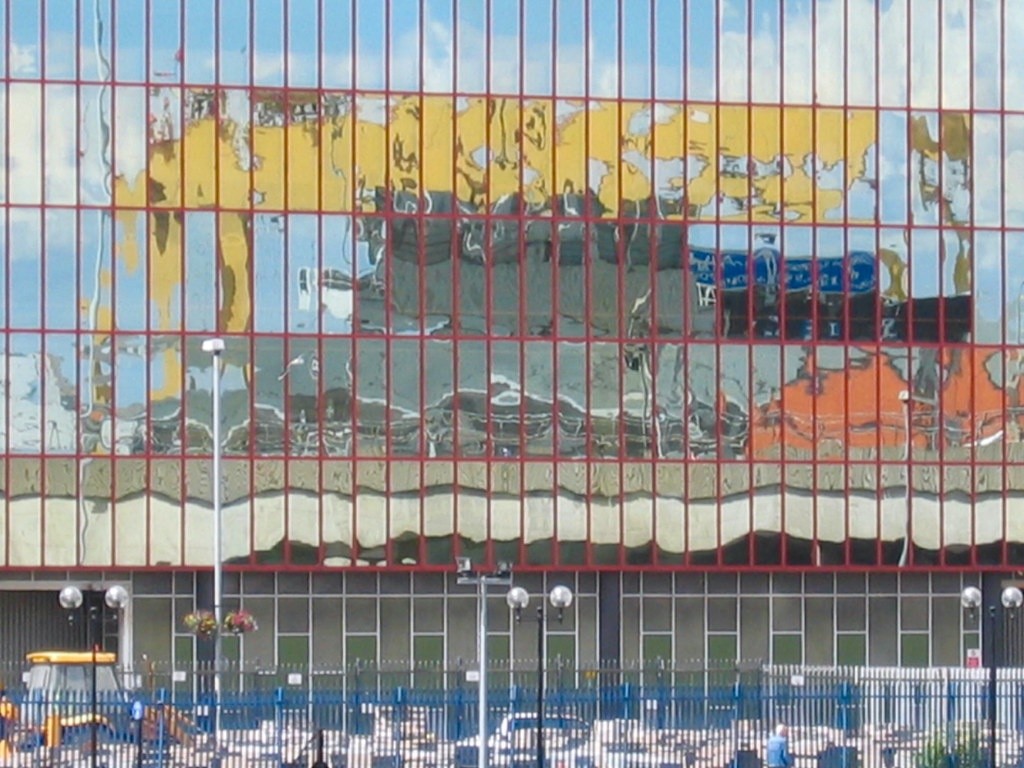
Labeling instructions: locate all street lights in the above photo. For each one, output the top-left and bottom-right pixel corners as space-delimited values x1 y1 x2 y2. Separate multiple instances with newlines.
960 585 1024 768
201 336 230 768
506 585 575 768
58 583 130 768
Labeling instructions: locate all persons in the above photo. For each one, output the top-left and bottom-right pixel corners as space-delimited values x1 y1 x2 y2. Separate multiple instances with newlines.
766 724 793 768
